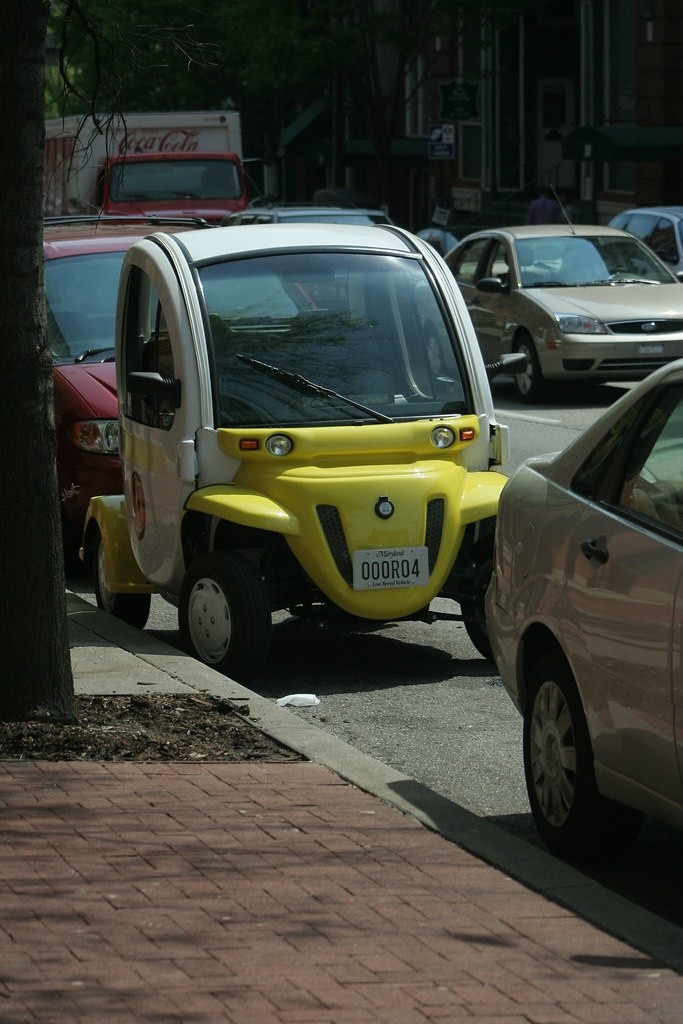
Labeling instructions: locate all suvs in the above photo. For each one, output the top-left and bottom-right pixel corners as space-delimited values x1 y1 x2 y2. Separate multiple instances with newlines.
43 216 222 578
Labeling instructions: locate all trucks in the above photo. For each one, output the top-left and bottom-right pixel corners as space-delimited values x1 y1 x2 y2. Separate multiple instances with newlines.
43 111 248 225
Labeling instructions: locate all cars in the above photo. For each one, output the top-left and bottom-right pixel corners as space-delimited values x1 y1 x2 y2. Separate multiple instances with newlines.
484 358 683 875
443 183 683 403
79 222 510 676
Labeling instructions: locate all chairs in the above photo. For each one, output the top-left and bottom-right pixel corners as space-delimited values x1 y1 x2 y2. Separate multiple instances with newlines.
208 313 233 336
291 310 354 337
497 245 544 288
